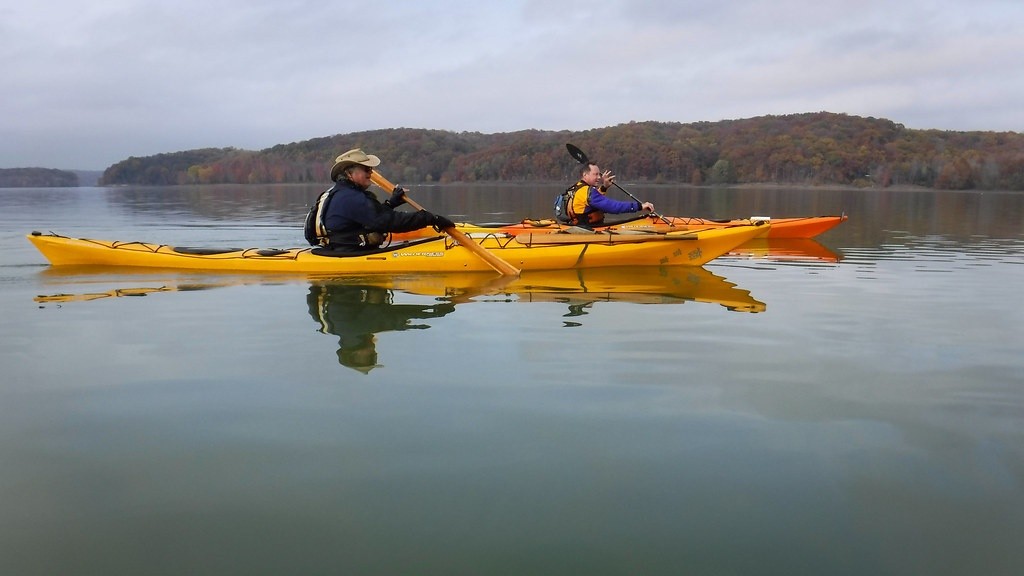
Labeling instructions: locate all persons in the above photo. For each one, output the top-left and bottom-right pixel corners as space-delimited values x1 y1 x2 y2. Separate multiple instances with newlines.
566 161 655 227
315 147 456 254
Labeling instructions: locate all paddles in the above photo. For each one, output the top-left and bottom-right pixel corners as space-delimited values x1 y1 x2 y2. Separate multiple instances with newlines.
565 141 673 228
368 166 521 277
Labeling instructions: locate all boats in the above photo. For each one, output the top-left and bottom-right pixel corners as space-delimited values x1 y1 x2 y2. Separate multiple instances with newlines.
24 222 770 272
388 216 847 239
31 267 768 315
726 239 843 262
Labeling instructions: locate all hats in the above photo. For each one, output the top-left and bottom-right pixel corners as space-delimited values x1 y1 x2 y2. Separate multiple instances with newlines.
330 148 381 182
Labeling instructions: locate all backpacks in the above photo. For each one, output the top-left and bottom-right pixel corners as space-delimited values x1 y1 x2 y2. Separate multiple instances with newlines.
554 183 590 222
303 186 378 247
